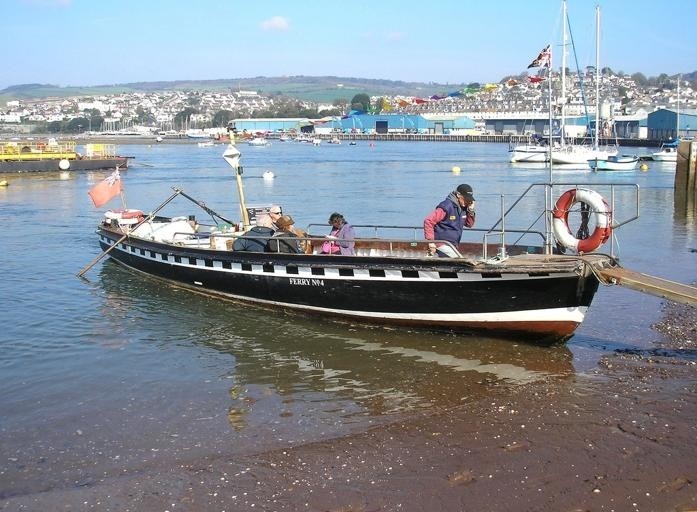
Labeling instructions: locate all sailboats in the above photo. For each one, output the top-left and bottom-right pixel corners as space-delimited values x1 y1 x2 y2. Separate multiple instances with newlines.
507 1 679 171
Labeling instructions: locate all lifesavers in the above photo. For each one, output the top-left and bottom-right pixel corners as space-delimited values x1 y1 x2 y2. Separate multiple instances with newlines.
102 208 145 225
551 188 613 255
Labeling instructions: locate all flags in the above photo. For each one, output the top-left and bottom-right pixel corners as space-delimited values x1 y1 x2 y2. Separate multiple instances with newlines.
87 174 120 208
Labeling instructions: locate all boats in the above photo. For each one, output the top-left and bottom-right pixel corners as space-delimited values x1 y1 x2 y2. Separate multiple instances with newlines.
1 139 128 173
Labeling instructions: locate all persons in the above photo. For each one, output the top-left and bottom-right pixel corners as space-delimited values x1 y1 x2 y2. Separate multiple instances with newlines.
320 213 355 255
424 185 475 259
231 206 313 255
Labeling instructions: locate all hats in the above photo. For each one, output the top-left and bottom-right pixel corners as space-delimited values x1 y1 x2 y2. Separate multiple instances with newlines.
276 215 294 227
456 183 474 202
